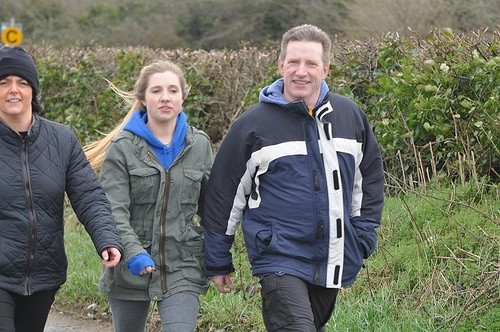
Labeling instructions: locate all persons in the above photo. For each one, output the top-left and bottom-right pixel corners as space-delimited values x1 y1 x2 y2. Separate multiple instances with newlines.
0 45 121 332
203 26 385 332
96 60 216 332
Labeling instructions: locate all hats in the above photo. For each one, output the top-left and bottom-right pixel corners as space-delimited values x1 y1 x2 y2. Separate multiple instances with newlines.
0 45 39 91
